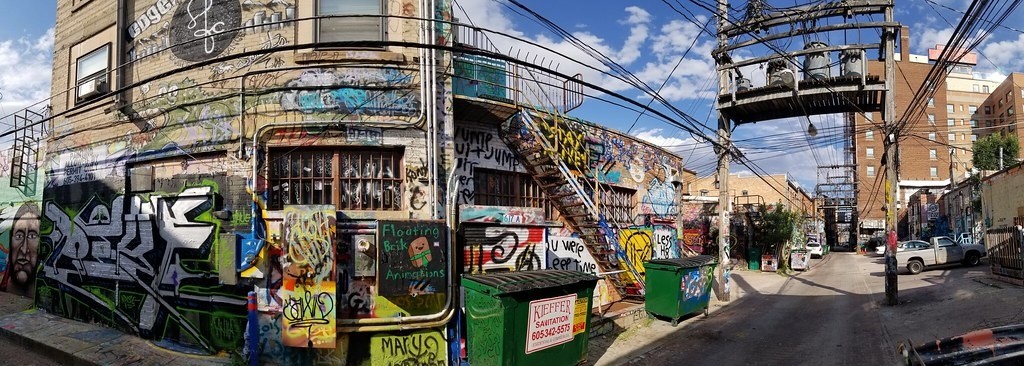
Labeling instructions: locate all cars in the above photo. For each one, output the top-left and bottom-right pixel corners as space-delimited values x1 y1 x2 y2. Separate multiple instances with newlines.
896 239 930 253
875 240 902 256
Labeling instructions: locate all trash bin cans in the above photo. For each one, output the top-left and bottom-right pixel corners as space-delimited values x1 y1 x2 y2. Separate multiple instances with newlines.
460 269 607 366
644 254 720 327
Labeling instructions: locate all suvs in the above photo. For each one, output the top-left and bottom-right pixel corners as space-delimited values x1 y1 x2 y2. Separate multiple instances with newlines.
805 242 823 259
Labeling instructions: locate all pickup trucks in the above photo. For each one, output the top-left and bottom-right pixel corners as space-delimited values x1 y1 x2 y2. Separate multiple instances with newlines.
895 236 987 275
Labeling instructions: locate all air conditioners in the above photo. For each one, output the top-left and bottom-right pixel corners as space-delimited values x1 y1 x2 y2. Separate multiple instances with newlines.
78 79 103 99
